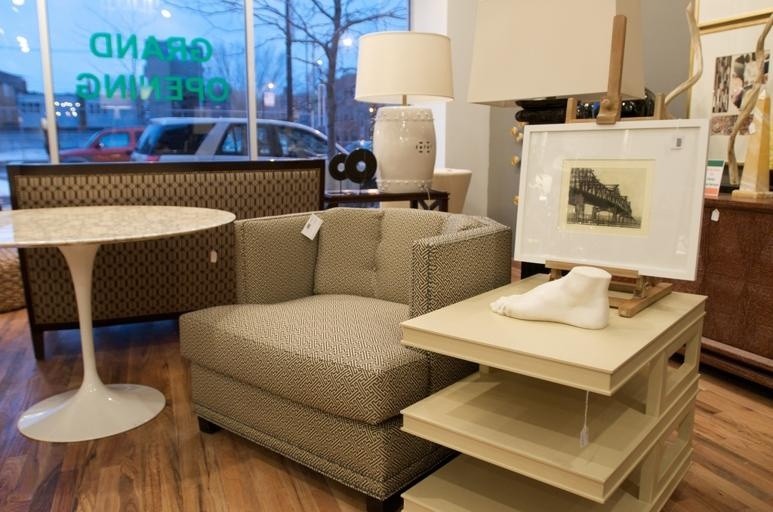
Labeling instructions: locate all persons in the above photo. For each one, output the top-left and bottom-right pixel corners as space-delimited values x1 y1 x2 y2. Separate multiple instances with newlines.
488 262 614 335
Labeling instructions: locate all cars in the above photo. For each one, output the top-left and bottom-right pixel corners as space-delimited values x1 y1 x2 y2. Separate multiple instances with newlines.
343 138 373 156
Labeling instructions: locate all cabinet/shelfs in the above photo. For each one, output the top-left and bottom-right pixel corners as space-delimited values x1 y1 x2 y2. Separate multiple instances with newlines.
399 267 708 511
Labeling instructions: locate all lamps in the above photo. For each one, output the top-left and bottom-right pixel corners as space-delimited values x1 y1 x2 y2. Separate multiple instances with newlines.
466 0 645 125
353 31 454 192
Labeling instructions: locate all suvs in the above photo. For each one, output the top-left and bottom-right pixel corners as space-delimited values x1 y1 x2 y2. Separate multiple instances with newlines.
57 125 146 168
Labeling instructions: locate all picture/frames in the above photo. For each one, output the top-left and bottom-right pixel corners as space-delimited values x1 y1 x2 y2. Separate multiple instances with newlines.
691 11 773 176
514 121 712 281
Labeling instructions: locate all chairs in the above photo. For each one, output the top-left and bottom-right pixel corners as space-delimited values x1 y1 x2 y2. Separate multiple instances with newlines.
177 205 511 509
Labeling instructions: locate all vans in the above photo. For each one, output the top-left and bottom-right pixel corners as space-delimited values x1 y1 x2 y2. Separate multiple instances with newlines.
131 112 376 212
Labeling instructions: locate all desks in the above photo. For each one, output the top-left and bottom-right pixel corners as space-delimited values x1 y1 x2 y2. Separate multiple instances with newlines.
0 205 235 444
320 189 449 211
